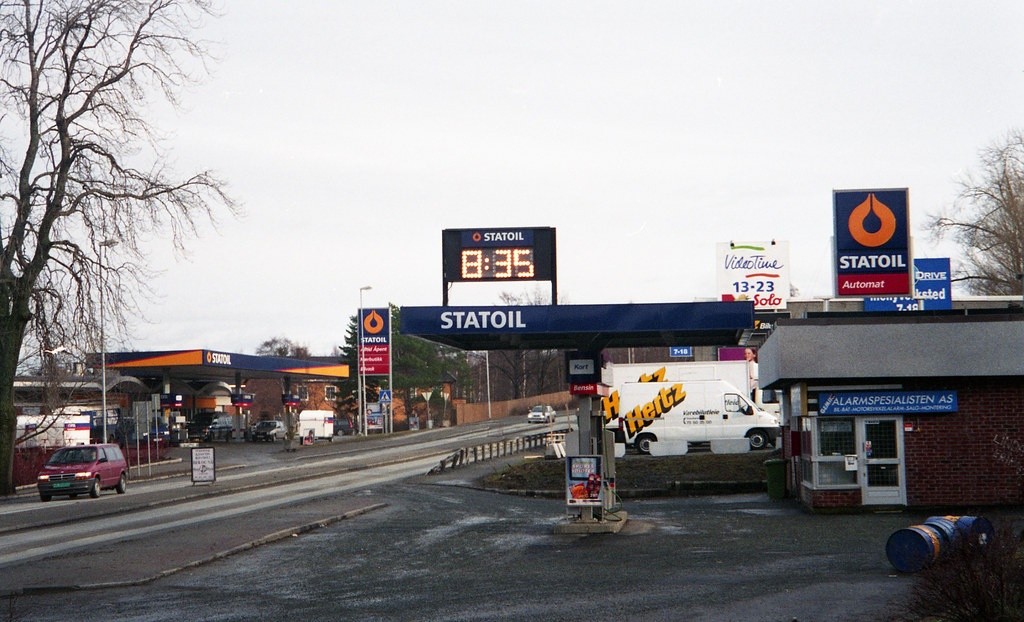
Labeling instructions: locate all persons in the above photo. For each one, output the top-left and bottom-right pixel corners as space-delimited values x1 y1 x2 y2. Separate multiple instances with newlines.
744 347 759 403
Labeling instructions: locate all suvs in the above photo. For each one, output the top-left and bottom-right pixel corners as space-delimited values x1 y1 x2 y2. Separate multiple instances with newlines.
184 411 233 442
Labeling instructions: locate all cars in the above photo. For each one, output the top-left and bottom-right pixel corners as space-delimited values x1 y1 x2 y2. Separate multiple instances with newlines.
528 406 557 424
252 420 287 442
333 418 353 437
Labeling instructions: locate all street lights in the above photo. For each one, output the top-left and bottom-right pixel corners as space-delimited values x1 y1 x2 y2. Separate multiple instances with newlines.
360 286 372 437
99 239 119 443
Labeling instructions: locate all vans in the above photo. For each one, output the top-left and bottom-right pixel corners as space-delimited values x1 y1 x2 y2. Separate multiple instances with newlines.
619 379 780 454
37 444 128 502
